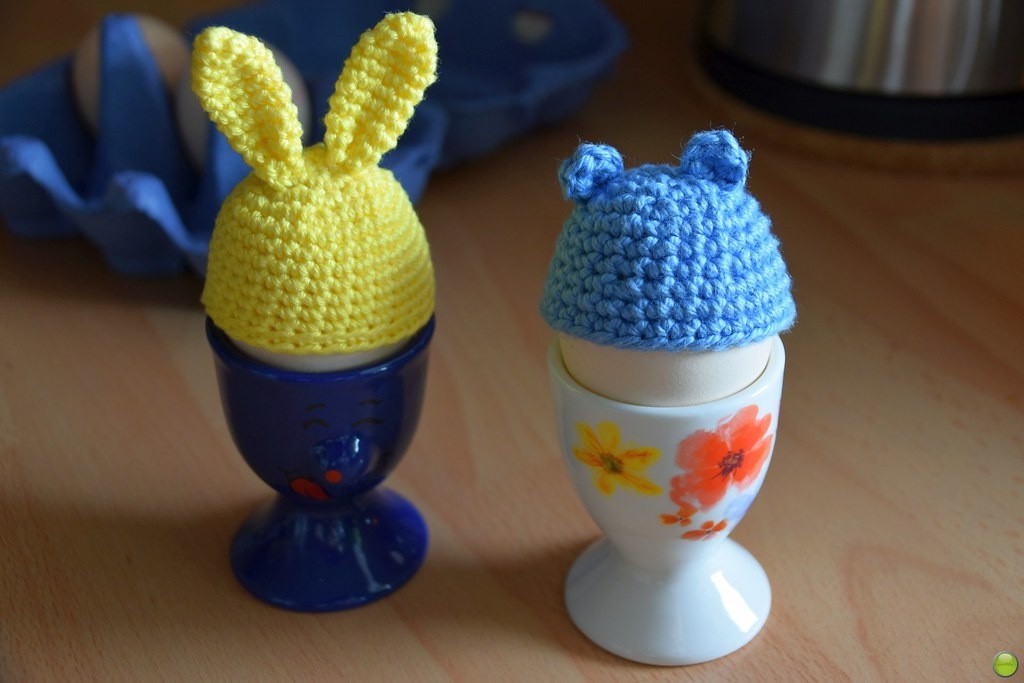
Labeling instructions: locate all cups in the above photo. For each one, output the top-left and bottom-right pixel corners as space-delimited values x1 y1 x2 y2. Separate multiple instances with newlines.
208 315 430 612
542 333 785 667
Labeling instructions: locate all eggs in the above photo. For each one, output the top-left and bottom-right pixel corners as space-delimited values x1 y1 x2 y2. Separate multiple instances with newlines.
560 164 772 407
210 141 435 371
